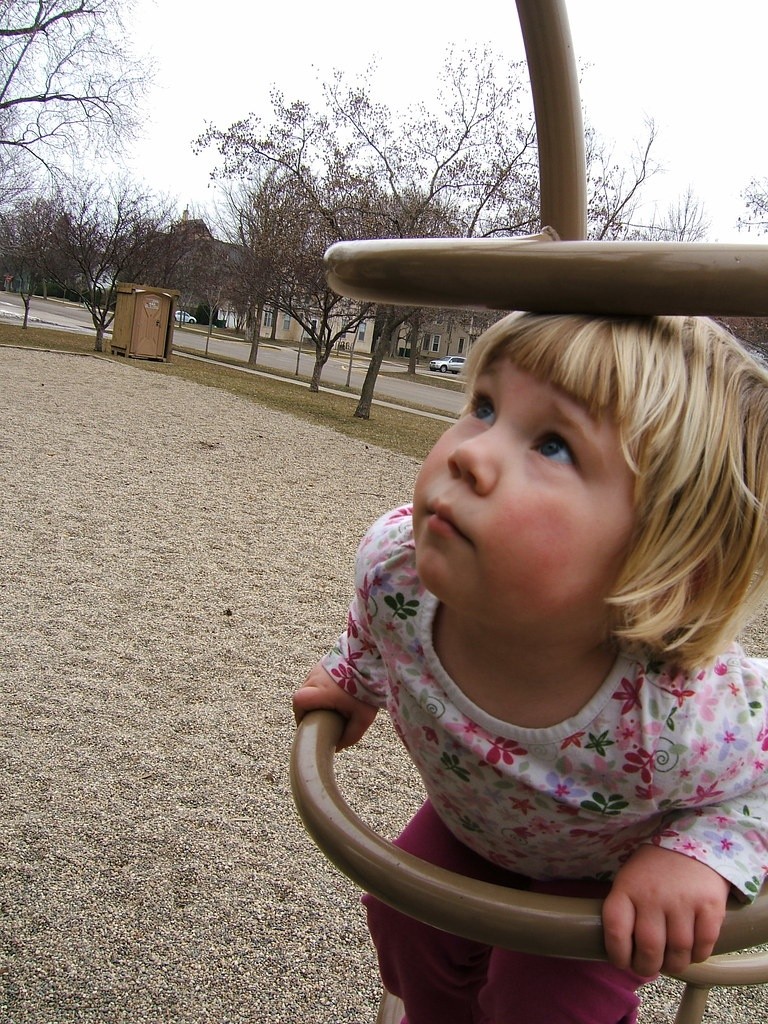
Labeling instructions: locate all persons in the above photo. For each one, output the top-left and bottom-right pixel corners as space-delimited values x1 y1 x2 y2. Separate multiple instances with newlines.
294 311 768 1024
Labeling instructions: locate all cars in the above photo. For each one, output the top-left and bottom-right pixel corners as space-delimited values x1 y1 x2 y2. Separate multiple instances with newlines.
174 311 197 324
429 356 466 373
